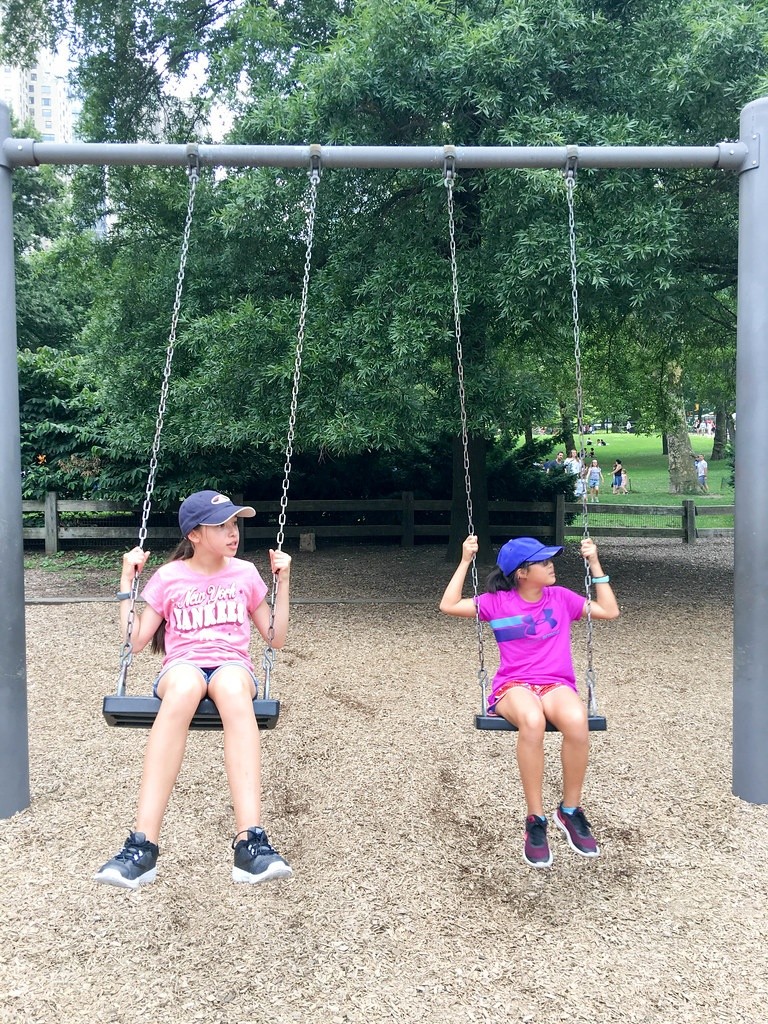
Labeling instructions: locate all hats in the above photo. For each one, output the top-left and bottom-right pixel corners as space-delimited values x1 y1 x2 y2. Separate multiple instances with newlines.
497 537 563 578
178 490 256 538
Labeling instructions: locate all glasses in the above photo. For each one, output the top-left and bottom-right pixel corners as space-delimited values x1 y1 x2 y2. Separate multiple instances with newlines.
519 557 555 569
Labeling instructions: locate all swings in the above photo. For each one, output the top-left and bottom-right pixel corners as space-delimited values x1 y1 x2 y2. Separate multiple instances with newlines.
442 166 610 731
103 169 323 728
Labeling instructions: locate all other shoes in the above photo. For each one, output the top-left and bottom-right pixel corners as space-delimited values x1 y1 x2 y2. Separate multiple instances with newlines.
591 496 599 502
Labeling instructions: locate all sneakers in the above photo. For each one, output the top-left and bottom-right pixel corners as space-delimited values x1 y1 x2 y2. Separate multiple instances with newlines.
554 801 601 859
93 827 161 890
522 814 554 867
230 825 294 884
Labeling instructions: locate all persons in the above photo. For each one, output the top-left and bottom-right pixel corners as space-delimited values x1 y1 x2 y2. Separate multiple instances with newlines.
693 419 716 436
537 437 629 503
438 533 620 868
693 454 710 494
626 421 632 434
95 489 295 890
582 424 593 434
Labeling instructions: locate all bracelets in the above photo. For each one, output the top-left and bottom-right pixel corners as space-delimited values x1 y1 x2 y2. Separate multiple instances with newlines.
592 574 610 585
114 585 137 603
